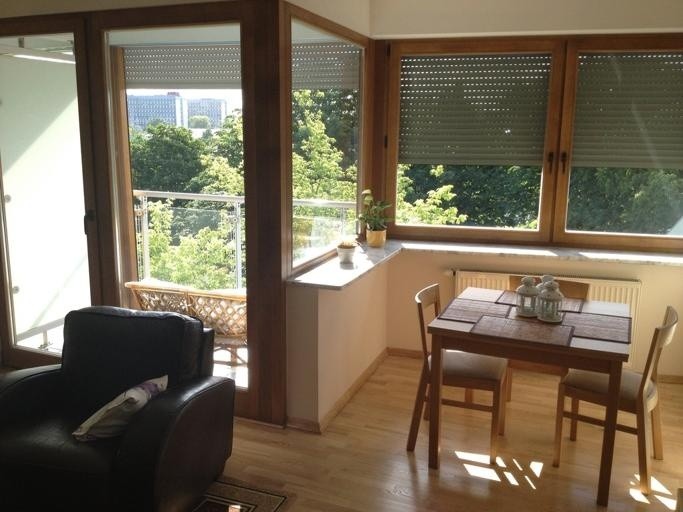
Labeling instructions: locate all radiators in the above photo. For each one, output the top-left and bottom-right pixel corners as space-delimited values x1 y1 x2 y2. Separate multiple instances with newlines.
443 265 641 369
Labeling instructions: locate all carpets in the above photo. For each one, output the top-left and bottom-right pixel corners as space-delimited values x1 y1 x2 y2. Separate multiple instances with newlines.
187 475 298 512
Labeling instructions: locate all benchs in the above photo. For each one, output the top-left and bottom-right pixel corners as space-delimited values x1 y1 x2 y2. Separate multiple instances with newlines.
124 282 248 367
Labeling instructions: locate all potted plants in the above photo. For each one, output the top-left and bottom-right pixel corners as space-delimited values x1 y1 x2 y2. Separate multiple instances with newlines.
336 241 356 264
354 189 399 247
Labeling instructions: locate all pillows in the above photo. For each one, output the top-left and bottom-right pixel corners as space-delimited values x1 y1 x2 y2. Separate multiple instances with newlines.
71 374 168 443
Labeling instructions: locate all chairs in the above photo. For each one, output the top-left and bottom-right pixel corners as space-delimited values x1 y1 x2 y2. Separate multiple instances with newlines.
503 275 589 402
551 305 679 495
406 283 508 465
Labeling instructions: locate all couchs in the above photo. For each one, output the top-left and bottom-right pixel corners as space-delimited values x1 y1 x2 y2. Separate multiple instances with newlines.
0 306 235 512
